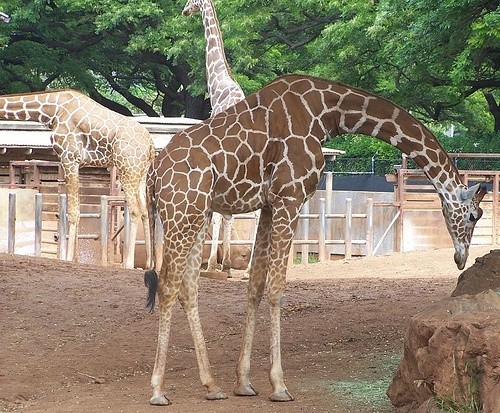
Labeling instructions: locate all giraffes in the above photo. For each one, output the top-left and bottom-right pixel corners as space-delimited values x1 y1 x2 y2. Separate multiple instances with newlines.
181 0 262 280
0 88 156 270
144 74 488 406
